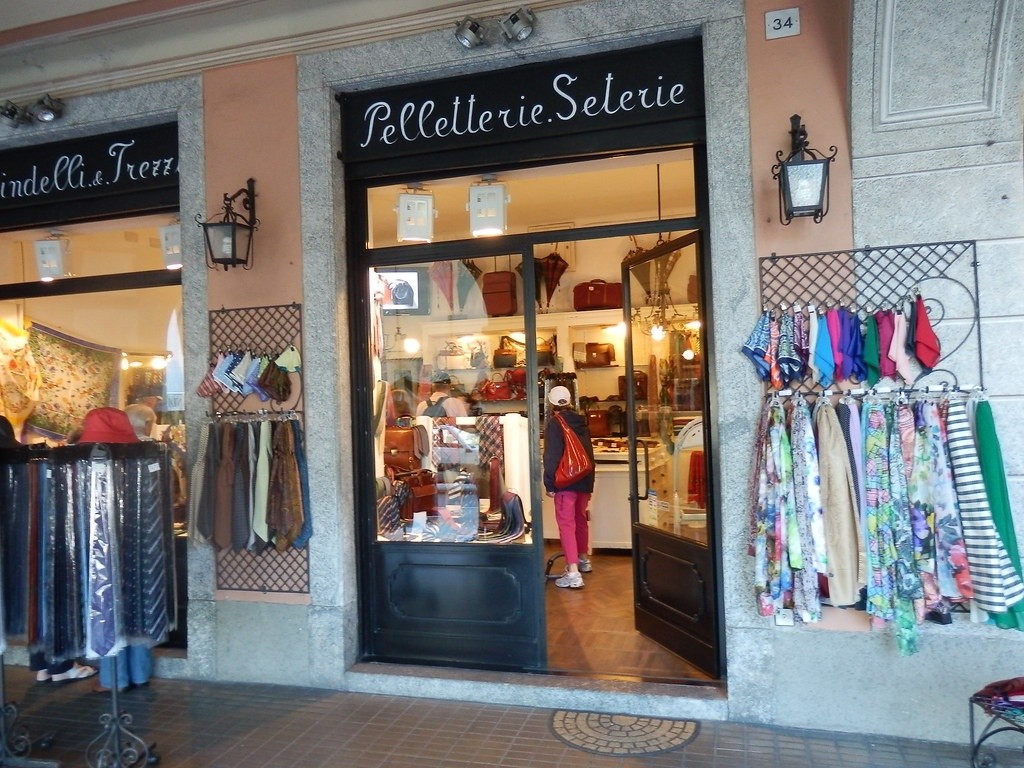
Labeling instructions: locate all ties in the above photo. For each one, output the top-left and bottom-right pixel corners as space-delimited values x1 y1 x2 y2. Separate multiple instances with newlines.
1 444 186 670
371 418 527 549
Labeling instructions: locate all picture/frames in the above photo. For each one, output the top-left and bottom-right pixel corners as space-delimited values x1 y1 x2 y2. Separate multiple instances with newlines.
527 222 577 272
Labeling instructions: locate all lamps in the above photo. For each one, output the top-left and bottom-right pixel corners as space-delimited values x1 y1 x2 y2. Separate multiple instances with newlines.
159 210 183 270
466 173 511 238
771 113 837 227
455 14 490 50
498 5 537 45
33 229 70 283
0 100 28 127
392 181 438 244
32 94 63 122
194 177 261 272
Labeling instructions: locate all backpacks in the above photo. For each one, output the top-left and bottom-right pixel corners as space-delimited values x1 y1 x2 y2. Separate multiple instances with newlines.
422 395 450 417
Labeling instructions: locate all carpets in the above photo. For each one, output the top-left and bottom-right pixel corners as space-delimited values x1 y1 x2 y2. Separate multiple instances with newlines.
550 708 700 756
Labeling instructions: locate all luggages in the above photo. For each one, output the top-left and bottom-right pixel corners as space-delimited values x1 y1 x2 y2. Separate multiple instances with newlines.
482 253 516 317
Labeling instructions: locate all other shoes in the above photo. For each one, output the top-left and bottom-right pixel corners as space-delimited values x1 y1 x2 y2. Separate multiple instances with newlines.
36 669 51 681
90 681 129 694
52 661 98 682
579 558 593 572
555 565 586 588
134 681 150 687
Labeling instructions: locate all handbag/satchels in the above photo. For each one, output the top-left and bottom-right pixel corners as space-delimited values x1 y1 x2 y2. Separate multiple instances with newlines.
618 370 648 401
375 329 535 418
585 403 653 440
400 469 439 519
547 411 593 489
661 343 703 411
383 417 422 473
576 342 611 369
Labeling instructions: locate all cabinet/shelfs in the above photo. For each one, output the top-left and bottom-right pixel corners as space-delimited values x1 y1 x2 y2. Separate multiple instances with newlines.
421 303 704 443
587 457 674 555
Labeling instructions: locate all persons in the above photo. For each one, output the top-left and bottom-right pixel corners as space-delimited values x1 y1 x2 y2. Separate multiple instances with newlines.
543 386 595 588
416 373 468 417
122 404 157 441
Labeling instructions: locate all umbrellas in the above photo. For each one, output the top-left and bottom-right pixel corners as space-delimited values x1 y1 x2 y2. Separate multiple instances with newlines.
429 259 483 315
515 241 570 314
623 231 682 298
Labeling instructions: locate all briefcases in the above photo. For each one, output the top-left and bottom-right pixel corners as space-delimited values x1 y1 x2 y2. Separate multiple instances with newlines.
572 278 624 311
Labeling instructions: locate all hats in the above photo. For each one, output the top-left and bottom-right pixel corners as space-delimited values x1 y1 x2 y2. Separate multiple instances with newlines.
427 372 452 384
